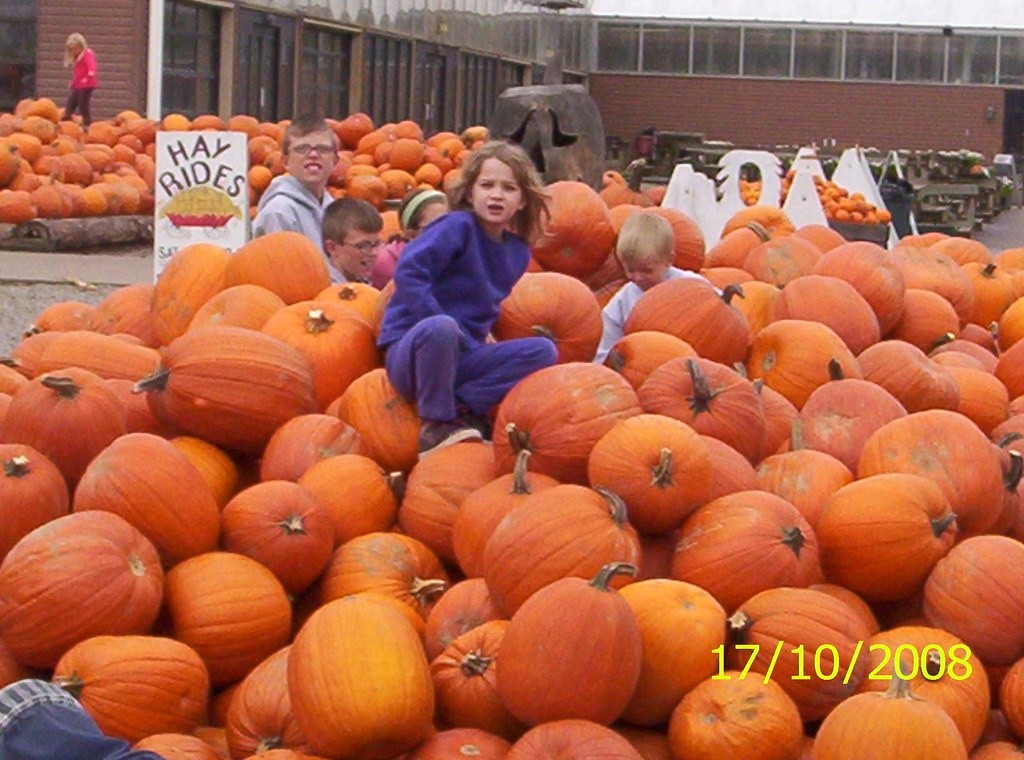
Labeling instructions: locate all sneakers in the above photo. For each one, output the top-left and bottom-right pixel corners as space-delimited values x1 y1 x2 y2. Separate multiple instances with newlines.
417 418 483 460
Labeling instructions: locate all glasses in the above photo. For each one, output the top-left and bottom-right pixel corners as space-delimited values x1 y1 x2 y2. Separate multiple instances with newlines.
344 239 386 254
287 144 337 157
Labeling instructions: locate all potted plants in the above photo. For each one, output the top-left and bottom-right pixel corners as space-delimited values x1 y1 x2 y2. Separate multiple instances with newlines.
637 126 657 156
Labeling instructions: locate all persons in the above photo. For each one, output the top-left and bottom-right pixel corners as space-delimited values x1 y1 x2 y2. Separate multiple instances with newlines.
0 679 165 760
591 212 725 366
252 111 558 462
60 33 98 133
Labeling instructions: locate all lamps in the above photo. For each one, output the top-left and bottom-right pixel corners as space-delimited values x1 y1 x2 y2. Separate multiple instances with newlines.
437 16 447 33
987 103 994 120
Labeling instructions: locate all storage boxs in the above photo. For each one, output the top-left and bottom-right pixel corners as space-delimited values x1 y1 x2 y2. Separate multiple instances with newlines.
993 154 1017 179
827 221 891 250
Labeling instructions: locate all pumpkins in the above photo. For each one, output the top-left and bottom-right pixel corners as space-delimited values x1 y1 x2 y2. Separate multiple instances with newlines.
0 96 1024 760
